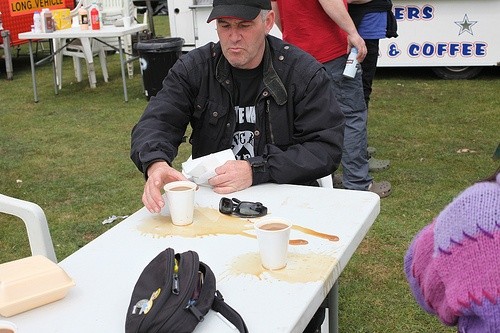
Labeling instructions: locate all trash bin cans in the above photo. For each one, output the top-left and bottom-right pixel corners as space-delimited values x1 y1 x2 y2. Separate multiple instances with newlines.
137 37 184 101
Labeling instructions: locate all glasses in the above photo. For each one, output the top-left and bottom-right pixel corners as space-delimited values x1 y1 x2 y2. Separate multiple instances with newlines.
219 197 268 218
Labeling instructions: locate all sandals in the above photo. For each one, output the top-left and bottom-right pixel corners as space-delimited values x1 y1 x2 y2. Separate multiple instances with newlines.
368 155 390 172
369 180 392 197
367 146 377 157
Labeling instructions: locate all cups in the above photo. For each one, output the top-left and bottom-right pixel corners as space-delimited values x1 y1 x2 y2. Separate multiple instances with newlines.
254 218 291 271
122 16 131 28
163 181 197 227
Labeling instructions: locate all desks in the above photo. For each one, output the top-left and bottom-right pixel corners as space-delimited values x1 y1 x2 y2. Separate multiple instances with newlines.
0 183 381 333
18 23 148 103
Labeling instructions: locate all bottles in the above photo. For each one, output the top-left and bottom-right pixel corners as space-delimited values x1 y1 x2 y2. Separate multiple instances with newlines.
78 5 89 30
33 7 53 34
342 47 359 79
88 3 102 30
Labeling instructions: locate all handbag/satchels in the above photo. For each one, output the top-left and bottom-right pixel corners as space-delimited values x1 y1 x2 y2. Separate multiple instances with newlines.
125 248 249 333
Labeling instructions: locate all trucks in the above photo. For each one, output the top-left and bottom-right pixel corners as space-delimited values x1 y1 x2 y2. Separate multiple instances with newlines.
166 0 500 81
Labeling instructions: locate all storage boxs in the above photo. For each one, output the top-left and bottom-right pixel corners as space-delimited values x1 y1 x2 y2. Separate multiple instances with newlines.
50 9 71 29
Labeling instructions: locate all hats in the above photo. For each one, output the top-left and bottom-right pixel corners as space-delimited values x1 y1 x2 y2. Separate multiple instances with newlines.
206 0 272 23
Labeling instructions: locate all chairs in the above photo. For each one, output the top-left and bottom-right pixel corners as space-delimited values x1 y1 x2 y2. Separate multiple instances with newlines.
0 194 58 264
180 156 333 333
54 0 137 91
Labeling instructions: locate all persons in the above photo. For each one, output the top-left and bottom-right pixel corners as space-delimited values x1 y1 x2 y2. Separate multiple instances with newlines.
404 142 500 333
270 0 392 200
347 0 397 172
131 0 346 333
